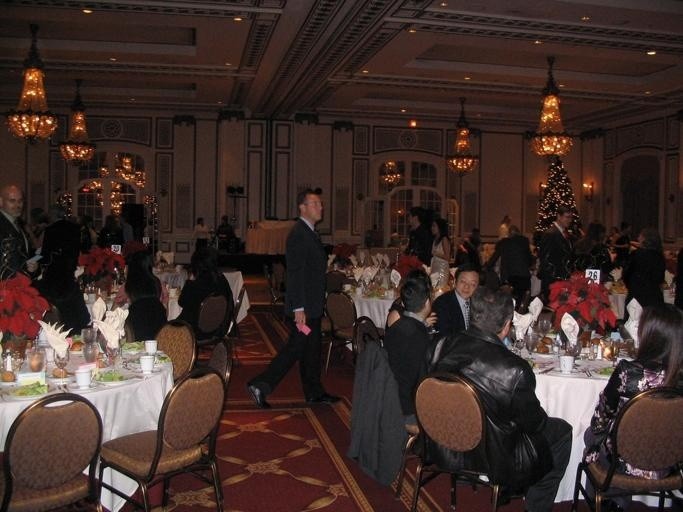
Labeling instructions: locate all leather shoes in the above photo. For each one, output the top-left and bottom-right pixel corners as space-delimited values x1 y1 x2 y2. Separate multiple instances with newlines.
247 382 270 409
305 394 342 404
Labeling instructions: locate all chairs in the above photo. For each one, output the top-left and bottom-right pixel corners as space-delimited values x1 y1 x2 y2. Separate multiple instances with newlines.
201 338 233 390
320 270 353 337
231 282 247 332
572 386 683 512
154 319 198 385
325 290 385 377
184 291 232 349
411 371 531 511
98 365 228 512
359 335 423 500
353 316 383 363
2 393 104 512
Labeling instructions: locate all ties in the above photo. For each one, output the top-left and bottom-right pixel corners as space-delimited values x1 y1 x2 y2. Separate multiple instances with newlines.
464 303 470 332
14 220 26 251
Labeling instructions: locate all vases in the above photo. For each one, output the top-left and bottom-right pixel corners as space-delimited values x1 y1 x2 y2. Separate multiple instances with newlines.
562 325 586 360
85 277 115 307
1 330 27 364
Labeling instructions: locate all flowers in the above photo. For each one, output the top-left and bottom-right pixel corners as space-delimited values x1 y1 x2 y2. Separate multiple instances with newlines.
74 246 126 312
545 272 617 362
0 271 52 367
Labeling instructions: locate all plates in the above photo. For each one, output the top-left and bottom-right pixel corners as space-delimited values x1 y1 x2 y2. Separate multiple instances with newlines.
5 394 49 404
95 379 137 387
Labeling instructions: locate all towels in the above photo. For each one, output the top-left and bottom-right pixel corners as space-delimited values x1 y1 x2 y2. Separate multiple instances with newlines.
364 266 380 281
430 272 439 287
371 256 379 267
352 268 363 282
422 264 432 276
37 320 73 358
376 253 383 264
624 316 639 348
664 270 674 285
104 307 129 340
391 269 401 288
93 317 119 351
348 254 357 266
383 254 388 263
528 297 544 321
360 252 364 263
626 297 643 320
92 297 106 320
512 310 533 340
560 311 580 346
328 255 336 265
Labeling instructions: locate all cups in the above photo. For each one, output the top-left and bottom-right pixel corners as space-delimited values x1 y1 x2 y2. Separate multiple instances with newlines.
524 333 539 362
140 355 155 374
356 288 363 296
565 340 581 358
95 287 105 302
74 369 92 390
105 341 120 373
81 328 97 345
144 341 157 354
538 319 552 341
84 347 100 362
53 348 69 386
559 355 574 376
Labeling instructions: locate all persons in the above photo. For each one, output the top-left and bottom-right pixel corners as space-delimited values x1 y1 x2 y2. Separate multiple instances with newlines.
384 278 433 418
621 227 666 325
97 215 123 249
584 303 683 512
175 249 235 341
191 218 212 251
78 215 96 254
384 269 439 332
35 205 78 302
539 206 577 305
455 228 482 272
24 207 51 258
0 184 42 284
403 206 434 267
431 265 479 338
570 223 611 282
487 225 532 312
606 226 618 244
471 270 515 348
246 187 342 409
612 221 633 267
429 218 450 301
432 285 573 512
216 215 233 251
499 217 511 238
109 252 170 319
121 221 132 240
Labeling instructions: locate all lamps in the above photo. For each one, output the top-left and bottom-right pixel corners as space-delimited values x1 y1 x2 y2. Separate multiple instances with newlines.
524 77 576 156
6 53 59 145
583 182 594 202
61 102 96 167
443 120 481 175
537 182 548 202
379 159 401 190
